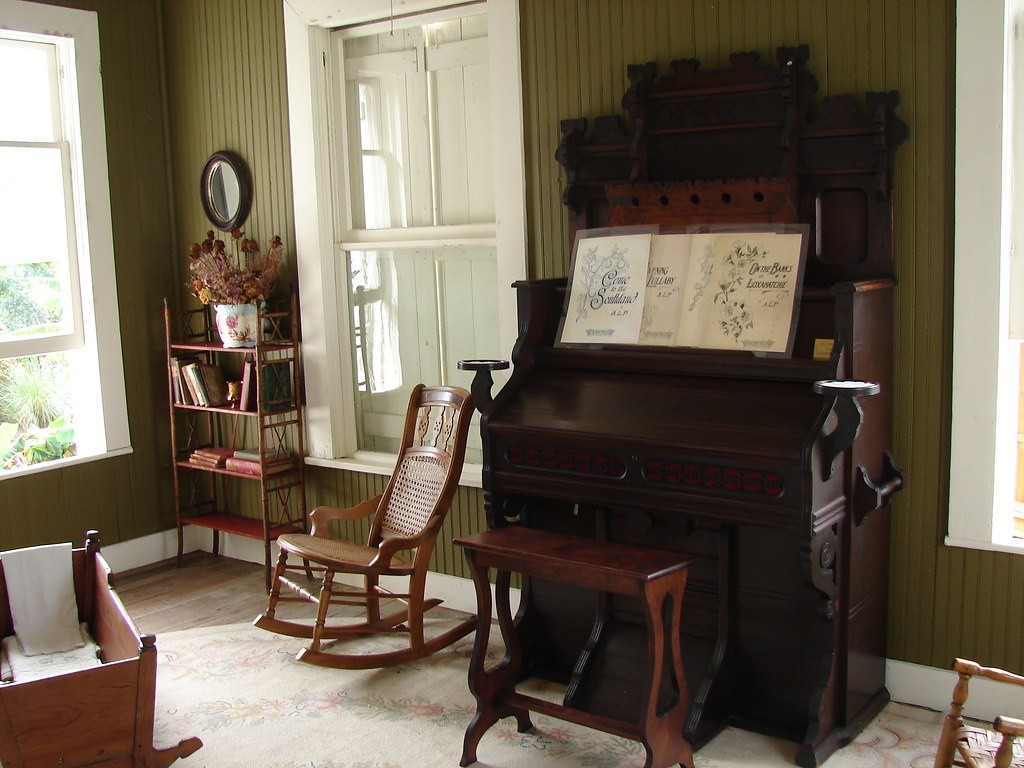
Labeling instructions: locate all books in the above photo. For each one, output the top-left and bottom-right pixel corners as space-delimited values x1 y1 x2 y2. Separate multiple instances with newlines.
189 447 233 468
241 361 291 411
171 355 228 408
226 447 294 476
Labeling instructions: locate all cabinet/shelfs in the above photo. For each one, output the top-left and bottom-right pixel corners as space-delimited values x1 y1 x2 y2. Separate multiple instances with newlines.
159 284 308 595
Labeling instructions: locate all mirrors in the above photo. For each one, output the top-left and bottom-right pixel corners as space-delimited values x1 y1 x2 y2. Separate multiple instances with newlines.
200 152 252 235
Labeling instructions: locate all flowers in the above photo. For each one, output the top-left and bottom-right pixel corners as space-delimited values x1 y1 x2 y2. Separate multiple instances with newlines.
181 231 283 307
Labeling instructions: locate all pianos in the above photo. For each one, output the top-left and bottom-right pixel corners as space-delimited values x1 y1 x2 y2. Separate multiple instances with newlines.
458 41 907 768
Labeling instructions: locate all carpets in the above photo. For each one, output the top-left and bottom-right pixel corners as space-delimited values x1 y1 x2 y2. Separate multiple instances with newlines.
139 612 967 768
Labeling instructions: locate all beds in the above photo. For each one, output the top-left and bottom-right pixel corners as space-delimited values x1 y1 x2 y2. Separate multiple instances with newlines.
0 528 204 768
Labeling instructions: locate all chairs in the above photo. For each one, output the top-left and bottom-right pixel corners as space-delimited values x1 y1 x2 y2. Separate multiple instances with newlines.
253 384 476 668
931 655 1024 768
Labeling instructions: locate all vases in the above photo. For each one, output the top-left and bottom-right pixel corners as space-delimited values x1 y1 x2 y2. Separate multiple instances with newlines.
214 300 270 350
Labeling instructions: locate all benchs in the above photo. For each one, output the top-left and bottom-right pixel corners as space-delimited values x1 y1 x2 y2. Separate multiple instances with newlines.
438 532 698 765
